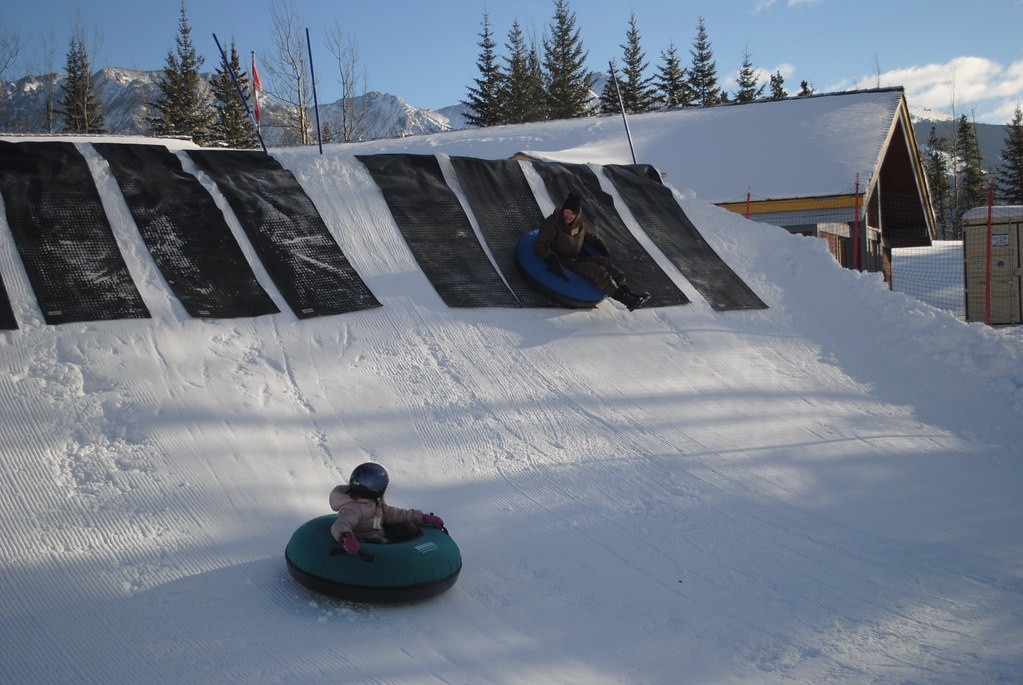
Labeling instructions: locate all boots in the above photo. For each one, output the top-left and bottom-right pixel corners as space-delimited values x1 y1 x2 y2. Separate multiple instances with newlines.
616 284 651 312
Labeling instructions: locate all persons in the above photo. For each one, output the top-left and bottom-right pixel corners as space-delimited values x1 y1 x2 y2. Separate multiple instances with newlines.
328 463 444 554
534 191 651 312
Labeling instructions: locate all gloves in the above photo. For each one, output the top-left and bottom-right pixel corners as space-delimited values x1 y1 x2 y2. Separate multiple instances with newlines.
424 513 443 529
341 530 360 555
542 251 561 269
592 234 609 256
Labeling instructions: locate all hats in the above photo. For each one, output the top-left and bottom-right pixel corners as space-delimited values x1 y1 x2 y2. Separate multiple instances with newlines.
563 193 582 214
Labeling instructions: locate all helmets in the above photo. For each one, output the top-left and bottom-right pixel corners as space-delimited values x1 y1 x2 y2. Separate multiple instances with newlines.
350 462 389 493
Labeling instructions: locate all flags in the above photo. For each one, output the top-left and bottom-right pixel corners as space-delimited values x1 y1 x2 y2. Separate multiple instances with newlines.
253 64 262 121
